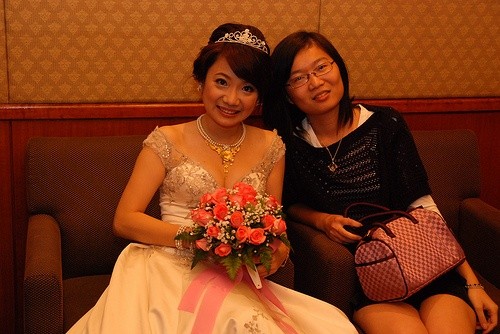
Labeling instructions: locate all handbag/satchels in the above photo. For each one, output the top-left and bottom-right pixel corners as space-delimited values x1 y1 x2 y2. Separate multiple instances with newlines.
343 202 466 303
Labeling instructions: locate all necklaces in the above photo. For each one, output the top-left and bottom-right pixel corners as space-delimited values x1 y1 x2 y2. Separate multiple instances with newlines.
197 114 246 174
308 117 346 172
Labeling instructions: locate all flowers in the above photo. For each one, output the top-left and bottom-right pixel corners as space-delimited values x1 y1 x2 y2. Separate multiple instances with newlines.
174 182 294 281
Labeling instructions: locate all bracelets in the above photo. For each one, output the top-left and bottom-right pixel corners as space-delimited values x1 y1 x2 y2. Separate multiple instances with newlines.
464 283 484 290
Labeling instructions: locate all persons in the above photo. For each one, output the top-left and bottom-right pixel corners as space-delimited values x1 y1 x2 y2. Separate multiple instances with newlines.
271 31 499 334
65 22 359 334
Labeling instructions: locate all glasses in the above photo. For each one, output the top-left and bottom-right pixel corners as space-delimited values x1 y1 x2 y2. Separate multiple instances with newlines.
286 60 336 89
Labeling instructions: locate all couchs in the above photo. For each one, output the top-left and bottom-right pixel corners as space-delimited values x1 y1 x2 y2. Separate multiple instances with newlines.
22 128 499 334
